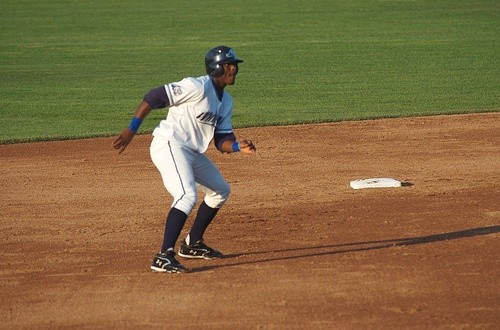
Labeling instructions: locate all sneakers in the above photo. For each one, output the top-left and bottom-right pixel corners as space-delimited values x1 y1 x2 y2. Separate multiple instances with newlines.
150 250 192 274
179 238 223 261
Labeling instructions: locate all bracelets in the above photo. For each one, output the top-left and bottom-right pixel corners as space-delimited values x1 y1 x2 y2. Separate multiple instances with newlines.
233 141 240 152
130 117 142 133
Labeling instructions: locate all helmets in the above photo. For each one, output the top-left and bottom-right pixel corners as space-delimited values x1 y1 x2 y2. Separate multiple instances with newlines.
204 45 244 77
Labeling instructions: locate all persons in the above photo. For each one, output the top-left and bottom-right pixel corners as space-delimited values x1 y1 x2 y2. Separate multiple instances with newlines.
112 46 256 274
